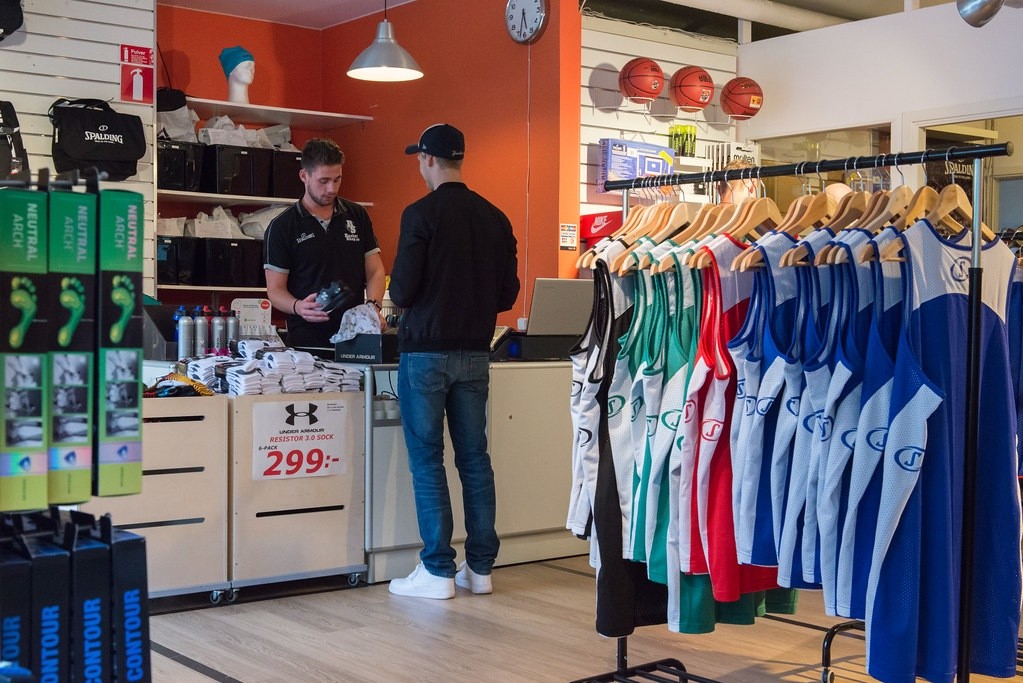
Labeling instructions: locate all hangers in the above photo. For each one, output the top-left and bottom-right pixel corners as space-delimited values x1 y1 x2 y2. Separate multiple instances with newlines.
574 146 1023 269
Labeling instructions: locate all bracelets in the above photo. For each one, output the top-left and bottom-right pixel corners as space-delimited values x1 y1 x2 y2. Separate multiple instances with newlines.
293 299 299 315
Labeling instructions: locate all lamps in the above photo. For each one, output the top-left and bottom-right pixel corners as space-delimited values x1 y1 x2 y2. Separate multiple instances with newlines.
346 0 424 82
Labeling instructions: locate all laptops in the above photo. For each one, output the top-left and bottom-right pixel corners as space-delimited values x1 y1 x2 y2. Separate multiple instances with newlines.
511 279 594 336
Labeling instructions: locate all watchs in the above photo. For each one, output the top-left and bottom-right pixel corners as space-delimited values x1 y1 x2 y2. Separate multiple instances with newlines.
366 299 382 311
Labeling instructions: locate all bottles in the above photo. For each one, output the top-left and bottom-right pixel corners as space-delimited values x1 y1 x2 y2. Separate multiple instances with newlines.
671 125 697 157
171 305 240 361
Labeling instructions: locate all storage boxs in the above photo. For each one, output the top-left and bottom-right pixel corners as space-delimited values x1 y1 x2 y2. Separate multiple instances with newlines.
334 332 400 365
598 138 675 195
0 166 153 683
157 138 306 199
142 304 217 361
156 235 266 287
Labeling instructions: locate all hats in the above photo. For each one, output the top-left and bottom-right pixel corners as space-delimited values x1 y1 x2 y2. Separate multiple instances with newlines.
218 46 254 80
405 123 465 161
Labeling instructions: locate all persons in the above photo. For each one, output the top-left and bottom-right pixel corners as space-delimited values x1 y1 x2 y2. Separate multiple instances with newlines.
388 123 520 599
219 46 255 104
261 137 385 346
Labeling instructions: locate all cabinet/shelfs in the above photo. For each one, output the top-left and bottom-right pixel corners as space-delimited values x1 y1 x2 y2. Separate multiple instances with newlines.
157 95 376 292
672 157 712 204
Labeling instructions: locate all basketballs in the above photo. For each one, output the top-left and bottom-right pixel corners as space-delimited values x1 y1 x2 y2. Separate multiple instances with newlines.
720 77 763 120
618 57 664 104
668 65 715 113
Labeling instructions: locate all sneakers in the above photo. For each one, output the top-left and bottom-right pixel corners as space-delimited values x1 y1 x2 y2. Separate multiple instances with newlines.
388 560 455 599
455 559 493 595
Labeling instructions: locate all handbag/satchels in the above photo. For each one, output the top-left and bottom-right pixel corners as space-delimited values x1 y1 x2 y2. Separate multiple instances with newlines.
0 0 24 43
47 98 147 183
0 100 32 189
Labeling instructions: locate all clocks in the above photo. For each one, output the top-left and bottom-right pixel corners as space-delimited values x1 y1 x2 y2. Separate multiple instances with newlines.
505 0 550 45
